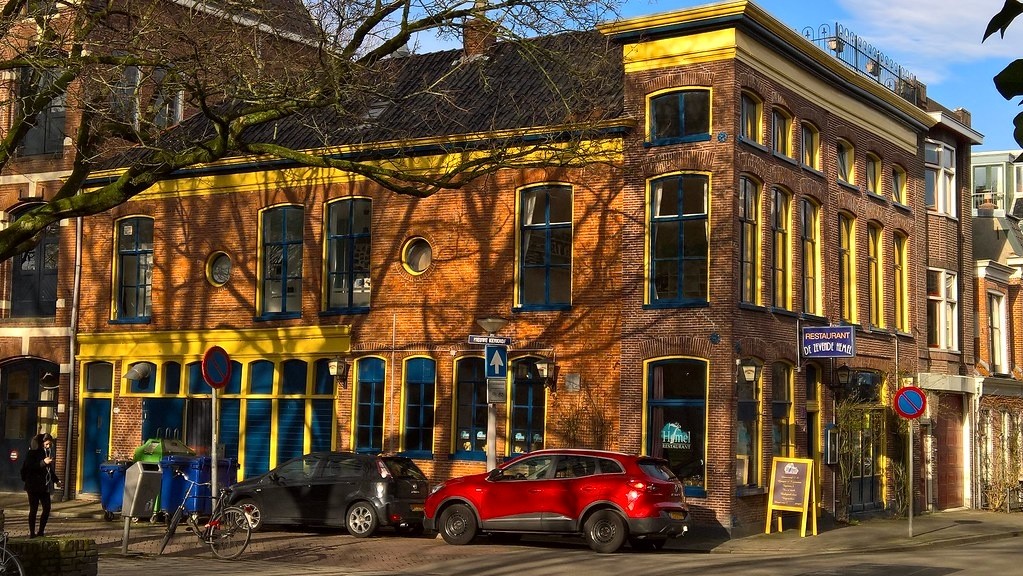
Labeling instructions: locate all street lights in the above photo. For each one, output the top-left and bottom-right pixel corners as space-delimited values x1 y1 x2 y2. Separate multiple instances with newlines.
475 313 509 472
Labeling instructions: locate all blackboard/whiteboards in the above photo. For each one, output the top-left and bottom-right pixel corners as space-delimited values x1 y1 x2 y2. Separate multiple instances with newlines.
768 457 817 512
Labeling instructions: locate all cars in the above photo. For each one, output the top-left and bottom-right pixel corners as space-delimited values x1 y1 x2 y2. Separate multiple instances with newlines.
425 447 689 555
222 450 429 537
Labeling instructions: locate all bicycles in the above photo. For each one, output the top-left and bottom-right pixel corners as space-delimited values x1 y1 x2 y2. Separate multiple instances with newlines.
0 530 27 576
157 469 255 559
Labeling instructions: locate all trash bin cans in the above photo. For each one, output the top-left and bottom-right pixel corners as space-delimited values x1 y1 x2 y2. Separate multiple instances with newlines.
120 461 162 522
160 455 209 526
203 453 240 521
132 438 197 523
99 458 132 521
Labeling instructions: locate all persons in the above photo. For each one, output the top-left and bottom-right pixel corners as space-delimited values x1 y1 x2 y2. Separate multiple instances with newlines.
20 433 62 538
